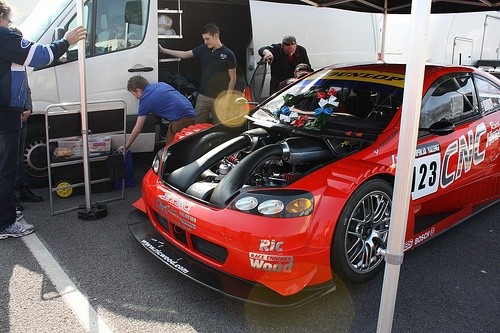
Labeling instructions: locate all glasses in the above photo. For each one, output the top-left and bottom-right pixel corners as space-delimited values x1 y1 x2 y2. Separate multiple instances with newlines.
283 41 295 46
4 13 13 26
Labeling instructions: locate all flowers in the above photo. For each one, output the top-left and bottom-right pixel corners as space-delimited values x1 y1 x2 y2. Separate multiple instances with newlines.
274 85 339 131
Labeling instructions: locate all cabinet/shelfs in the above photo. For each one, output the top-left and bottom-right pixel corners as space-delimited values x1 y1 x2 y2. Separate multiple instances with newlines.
157 0 184 39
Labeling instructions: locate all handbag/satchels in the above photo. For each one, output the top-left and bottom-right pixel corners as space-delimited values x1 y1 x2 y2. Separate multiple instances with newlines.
106 149 135 189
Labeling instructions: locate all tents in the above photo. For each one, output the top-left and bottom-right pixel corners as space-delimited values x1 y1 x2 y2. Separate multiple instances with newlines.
76 0 500 333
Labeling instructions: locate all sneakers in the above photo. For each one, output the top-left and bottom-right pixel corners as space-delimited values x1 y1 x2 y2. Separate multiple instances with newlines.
15 211 23 221
0 222 35 240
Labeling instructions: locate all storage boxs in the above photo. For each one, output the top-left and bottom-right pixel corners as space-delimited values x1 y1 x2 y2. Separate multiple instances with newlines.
111 133 160 153
58 137 82 149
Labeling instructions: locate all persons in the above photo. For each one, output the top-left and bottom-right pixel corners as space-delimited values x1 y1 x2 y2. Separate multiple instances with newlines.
258 35 314 95
158 23 238 125
0 1 88 240
117 76 197 153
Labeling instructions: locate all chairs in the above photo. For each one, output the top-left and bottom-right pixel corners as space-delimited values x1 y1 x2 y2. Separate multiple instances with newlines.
96 14 115 51
345 91 373 118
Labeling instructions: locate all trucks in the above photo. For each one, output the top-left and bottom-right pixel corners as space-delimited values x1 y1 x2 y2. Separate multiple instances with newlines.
18 0 500 187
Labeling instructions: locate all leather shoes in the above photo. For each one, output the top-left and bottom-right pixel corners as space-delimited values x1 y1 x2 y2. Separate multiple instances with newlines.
15 202 23 210
19 188 42 202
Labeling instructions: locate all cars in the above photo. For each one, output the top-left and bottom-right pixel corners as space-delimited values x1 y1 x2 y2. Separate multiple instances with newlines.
126 60 500 310
